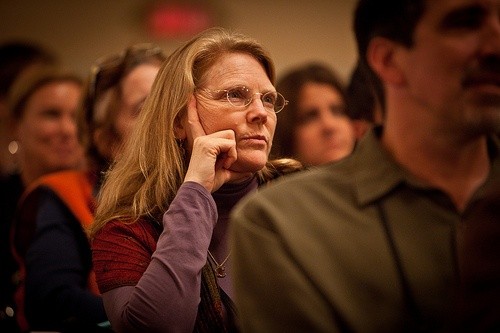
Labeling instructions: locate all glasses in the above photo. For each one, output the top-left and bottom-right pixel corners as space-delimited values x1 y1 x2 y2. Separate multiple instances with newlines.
204 86 289 113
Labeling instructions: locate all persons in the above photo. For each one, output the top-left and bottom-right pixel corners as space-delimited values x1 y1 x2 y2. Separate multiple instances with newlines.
90 27 287 333
2 1 499 332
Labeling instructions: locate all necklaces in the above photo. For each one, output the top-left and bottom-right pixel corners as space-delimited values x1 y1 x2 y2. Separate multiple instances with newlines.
206 248 233 278
